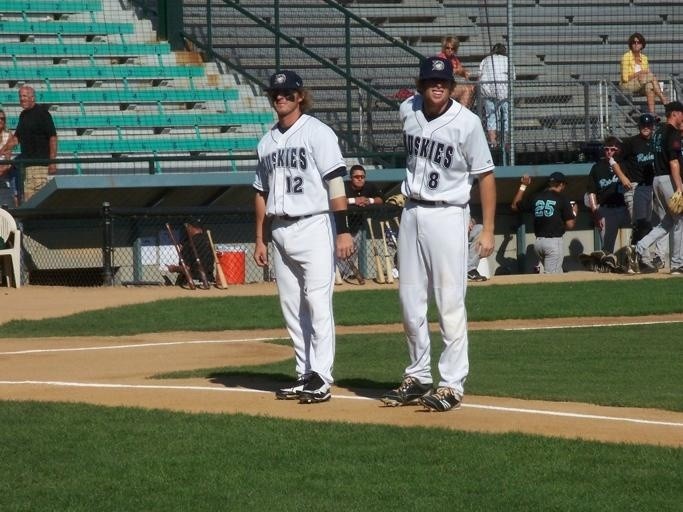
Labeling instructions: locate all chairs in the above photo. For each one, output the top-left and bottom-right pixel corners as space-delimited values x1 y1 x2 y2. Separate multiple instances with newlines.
0 204 25 288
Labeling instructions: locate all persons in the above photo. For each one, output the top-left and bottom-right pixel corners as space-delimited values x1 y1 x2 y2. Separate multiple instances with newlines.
618 32 669 123
0 109 14 176
343 165 385 282
383 57 497 411
253 70 355 405
434 34 473 107
168 217 214 286
478 43 515 147
0 87 57 207
467 219 487 282
586 101 683 273
510 172 578 274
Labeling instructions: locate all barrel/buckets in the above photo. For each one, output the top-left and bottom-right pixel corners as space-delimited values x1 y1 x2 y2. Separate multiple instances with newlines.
214 246 247 285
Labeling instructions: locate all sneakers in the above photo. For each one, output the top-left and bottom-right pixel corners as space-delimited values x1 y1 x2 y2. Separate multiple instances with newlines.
274 372 331 404
640 254 665 273
419 385 463 411
669 264 683 274
380 375 434 406
626 245 639 274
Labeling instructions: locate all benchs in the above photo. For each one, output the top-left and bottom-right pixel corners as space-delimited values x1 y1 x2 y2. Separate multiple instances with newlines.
1 0 682 167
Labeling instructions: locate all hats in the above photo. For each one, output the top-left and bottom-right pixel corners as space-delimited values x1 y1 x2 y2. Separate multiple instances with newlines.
546 171 568 183
637 113 654 125
419 55 453 81
262 70 303 93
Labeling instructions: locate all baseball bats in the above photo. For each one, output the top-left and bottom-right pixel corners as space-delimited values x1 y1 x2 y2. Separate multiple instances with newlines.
366 216 400 285
206 230 231 290
184 222 211 290
165 223 197 290
346 257 366 285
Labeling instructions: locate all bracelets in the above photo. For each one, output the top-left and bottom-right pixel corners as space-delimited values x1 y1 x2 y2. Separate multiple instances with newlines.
349 198 355 204
369 198 375 205
572 210 576 216
520 184 527 191
332 210 352 235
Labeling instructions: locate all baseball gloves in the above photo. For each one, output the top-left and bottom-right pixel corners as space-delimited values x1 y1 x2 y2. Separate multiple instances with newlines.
668 192 683 216
